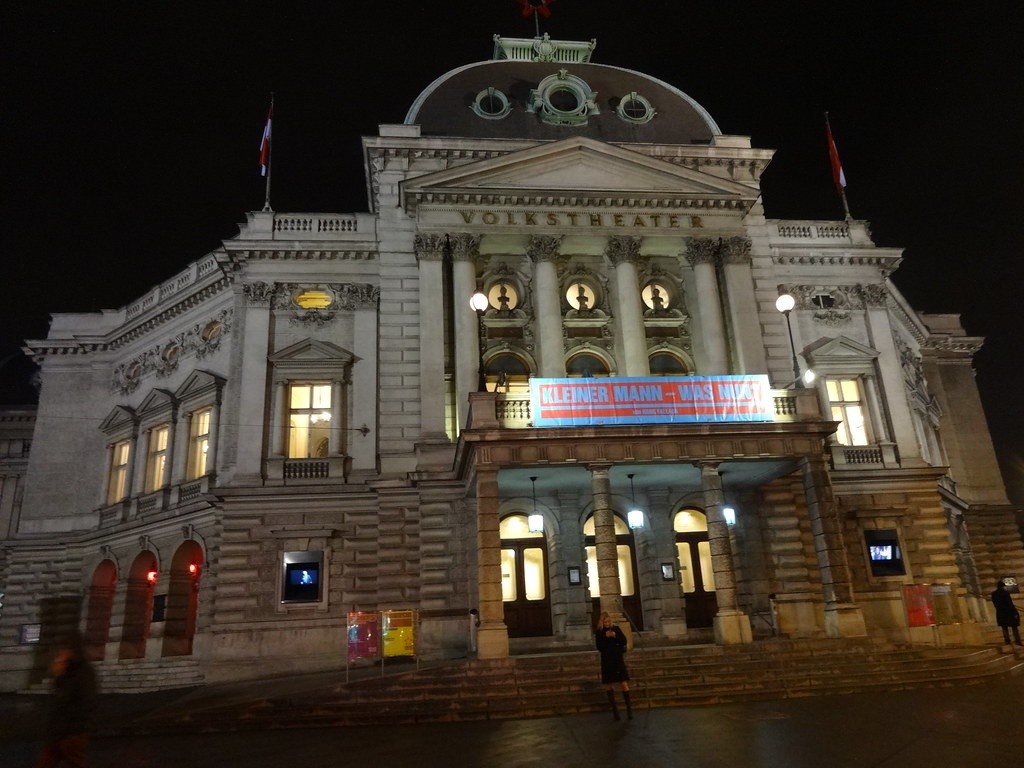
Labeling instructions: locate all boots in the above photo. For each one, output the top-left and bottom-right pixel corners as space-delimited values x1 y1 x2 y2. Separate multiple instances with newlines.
606 689 620 721
621 690 633 719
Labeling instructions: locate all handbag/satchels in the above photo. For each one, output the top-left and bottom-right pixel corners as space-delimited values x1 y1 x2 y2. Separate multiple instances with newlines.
612 624 627 654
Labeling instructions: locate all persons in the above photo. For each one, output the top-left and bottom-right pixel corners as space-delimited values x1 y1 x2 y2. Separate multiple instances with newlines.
826 121 848 192
37 631 99 767
595 612 635 720
991 581 1024 646
259 101 272 176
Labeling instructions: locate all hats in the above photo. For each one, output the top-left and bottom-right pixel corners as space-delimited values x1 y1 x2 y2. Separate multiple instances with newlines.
997 581 1006 588
